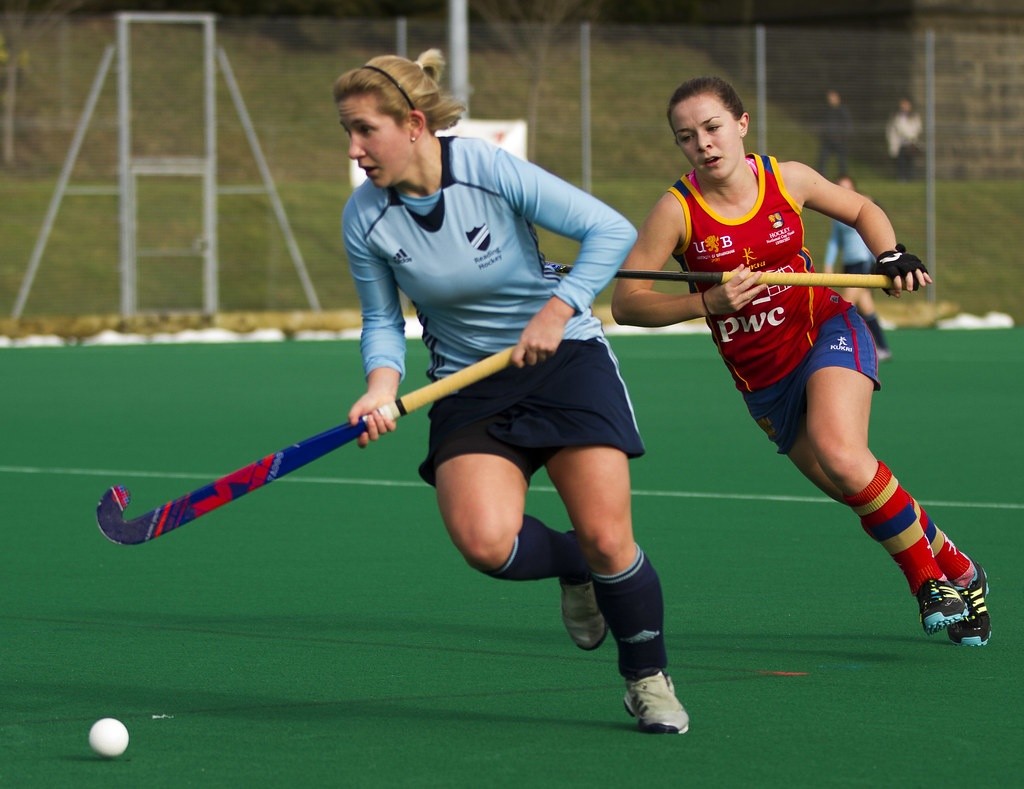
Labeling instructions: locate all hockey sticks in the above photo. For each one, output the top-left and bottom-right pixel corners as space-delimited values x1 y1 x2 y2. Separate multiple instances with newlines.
544 260 920 293
95 343 526 547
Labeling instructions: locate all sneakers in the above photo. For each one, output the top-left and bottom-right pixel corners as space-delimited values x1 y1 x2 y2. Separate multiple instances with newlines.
559 529 608 651
917 580 970 636
624 672 688 733
947 554 992 646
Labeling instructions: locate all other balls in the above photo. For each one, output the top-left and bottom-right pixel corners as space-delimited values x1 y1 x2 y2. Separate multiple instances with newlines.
88 718 130 758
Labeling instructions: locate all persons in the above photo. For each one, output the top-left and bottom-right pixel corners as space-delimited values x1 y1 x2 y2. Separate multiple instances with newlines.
884 94 924 181
334 49 690 735
611 73 994 646
823 174 892 362
810 81 859 193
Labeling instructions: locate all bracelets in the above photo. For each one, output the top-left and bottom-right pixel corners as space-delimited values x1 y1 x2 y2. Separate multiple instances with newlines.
701 292 711 316
